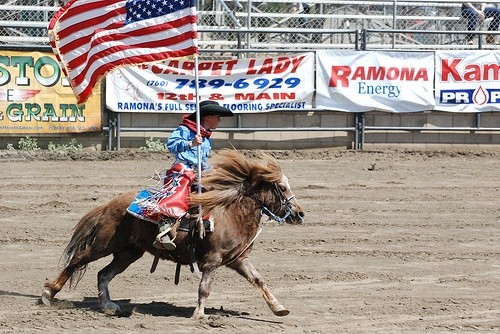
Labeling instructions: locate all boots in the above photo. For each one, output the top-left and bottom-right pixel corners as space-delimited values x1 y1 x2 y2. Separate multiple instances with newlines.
152 218 176 250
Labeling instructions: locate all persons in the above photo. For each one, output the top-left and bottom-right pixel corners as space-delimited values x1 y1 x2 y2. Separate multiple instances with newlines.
201 0 330 43
460 2 500 44
153 100 235 250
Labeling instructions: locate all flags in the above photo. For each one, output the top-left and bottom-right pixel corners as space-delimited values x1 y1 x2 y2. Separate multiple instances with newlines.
47 0 199 106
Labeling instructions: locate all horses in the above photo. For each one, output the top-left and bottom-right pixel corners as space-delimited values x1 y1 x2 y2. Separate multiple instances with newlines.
41 149 305 319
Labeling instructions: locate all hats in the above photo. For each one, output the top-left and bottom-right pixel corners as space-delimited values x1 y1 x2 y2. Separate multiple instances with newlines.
184 101 234 121
